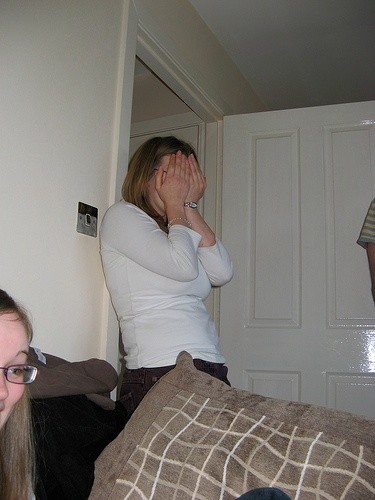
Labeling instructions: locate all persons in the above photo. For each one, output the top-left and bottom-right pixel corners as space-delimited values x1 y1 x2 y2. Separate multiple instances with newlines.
357 198 375 302
99 135 234 433
0 289 35 500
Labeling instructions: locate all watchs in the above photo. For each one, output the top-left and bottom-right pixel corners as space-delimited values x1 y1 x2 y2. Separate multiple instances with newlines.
184 202 197 208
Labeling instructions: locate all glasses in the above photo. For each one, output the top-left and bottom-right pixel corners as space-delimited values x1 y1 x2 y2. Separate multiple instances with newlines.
0 364 38 384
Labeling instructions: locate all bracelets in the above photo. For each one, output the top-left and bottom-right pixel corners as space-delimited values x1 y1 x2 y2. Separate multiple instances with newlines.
168 218 192 227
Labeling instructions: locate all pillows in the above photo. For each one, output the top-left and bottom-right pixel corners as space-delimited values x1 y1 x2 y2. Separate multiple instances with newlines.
85 351 375 500
